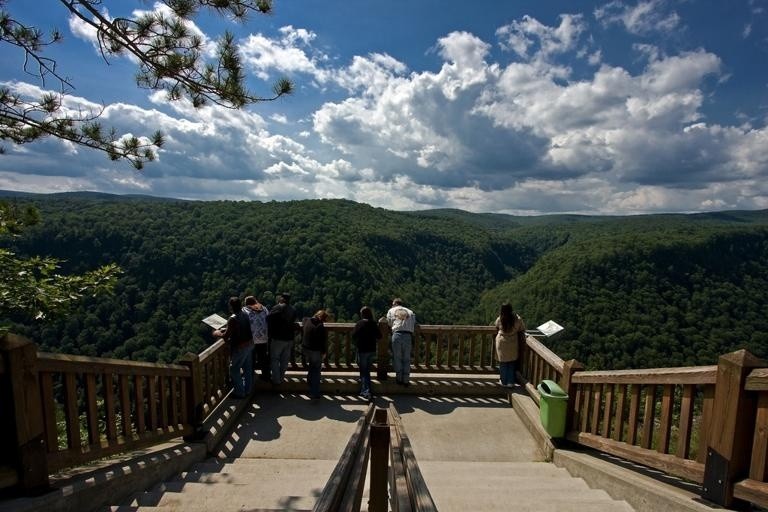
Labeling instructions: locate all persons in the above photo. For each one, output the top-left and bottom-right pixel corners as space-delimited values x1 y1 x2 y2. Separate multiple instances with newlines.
386 297 416 388
212 296 256 400
300 309 329 399
241 295 272 381
351 306 382 400
266 293 297 386
494 302 525 387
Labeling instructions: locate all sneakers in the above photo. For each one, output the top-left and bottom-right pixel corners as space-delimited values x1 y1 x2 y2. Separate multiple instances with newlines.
499 379 515 387
396 378 409 387
229 379 284 399
311 393 322 399
359 391 372 398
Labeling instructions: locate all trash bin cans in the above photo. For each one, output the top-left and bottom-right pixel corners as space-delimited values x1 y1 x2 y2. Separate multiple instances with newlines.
537 379 569 438
